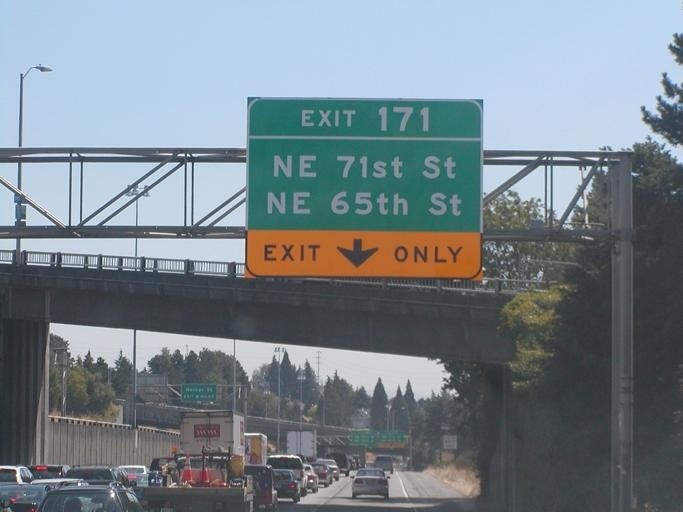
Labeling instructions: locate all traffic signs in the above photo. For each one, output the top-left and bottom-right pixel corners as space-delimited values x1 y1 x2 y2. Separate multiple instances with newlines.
244 97 482 281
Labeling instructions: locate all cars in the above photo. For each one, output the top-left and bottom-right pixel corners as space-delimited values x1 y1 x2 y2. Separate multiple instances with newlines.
302 464 320 492
351 468 391 499
274 469 302 502
0 410 277 512
316 459 341 483
312 463 333 488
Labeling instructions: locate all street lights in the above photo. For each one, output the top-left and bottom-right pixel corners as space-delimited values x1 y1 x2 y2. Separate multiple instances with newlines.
126 185 151 429
296 376 306 430
274 347 284 454
15 64 53 266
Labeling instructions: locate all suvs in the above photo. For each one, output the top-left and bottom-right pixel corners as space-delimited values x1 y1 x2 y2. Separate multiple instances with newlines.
326 452 351 477
374 456 395 474
36 480 146 512
66 464 130 487
266 454 310 498
